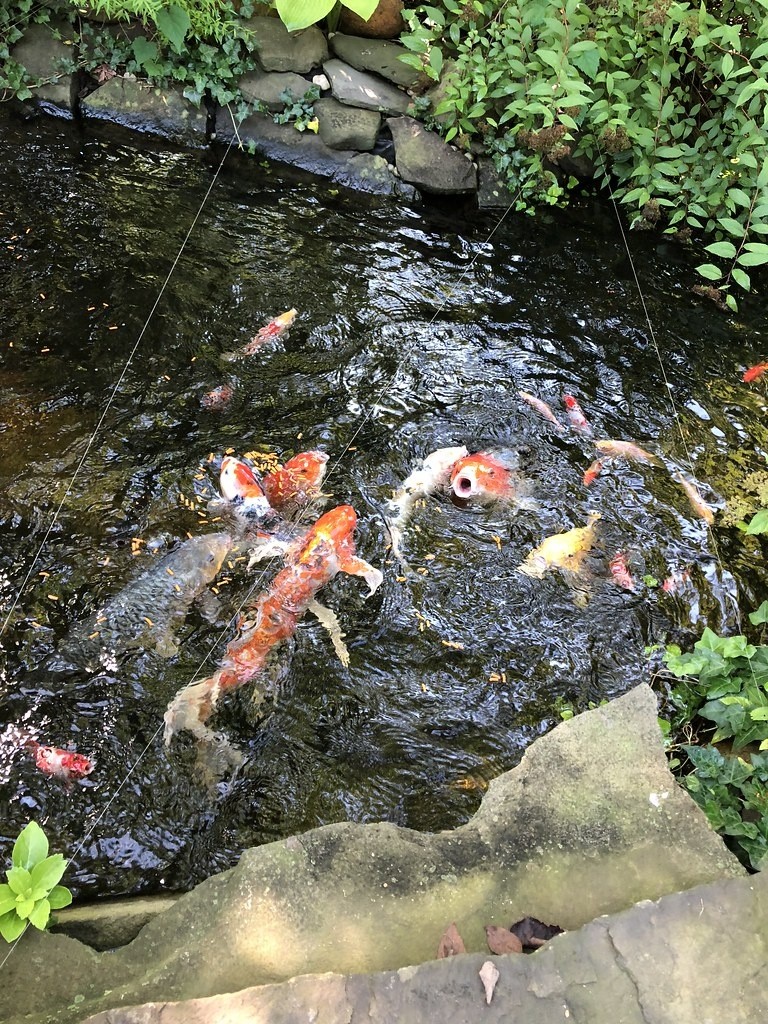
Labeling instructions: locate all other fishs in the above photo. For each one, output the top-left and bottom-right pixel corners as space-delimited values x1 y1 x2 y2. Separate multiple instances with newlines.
22 305 768 798
